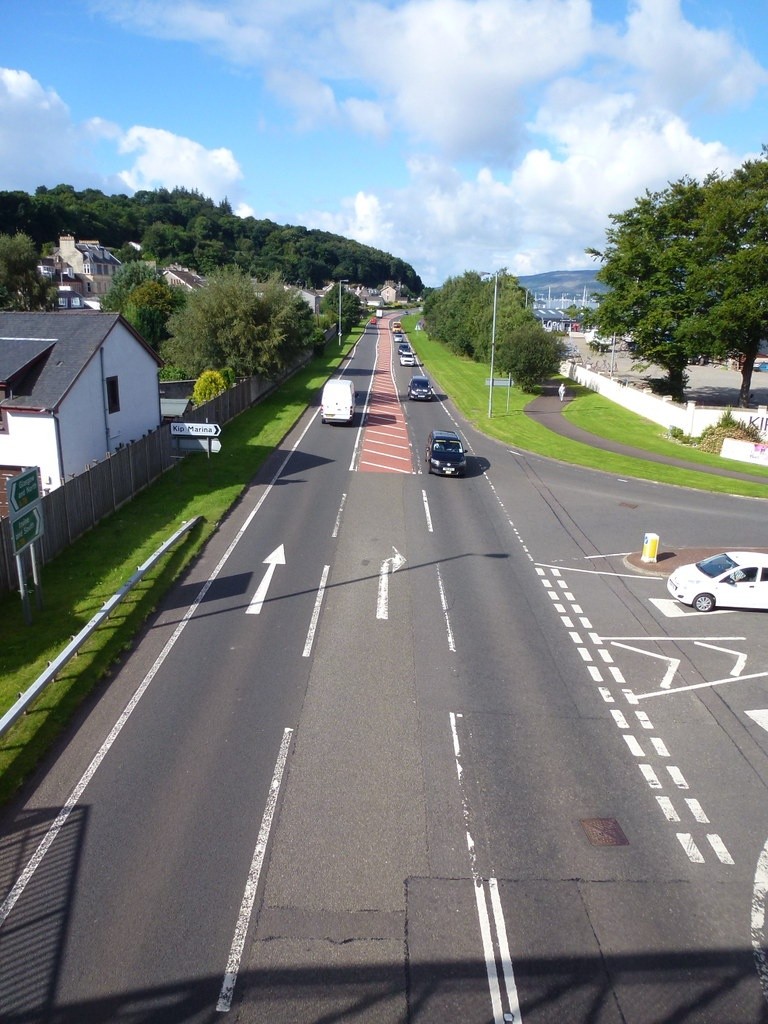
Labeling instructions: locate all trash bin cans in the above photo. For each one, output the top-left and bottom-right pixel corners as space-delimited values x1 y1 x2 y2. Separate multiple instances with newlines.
641 532 659 564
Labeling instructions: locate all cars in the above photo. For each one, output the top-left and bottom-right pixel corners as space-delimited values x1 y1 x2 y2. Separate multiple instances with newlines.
400 352 416 367
404 310 408 315
407 376 434 400
425 430 468 476
394 334 403 342
398 343 411 355
667 551 768 612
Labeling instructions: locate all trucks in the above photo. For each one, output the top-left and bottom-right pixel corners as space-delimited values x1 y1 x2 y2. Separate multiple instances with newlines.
392 322 401 333
320 379 359 424
377 310 382 318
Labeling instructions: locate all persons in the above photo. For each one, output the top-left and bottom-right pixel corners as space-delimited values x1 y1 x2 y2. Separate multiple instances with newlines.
434 443 444 450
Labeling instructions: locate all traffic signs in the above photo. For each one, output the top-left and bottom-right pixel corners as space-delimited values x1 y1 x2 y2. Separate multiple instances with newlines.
170 422 222 437
8 503 45 556
4 465 41 521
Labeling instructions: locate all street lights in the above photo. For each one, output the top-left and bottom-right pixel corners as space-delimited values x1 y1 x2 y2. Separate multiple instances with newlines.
339 280 349 345
481 271 497 418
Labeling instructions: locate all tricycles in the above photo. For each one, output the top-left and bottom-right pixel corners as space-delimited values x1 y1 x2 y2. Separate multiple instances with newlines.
370 316 377 324
